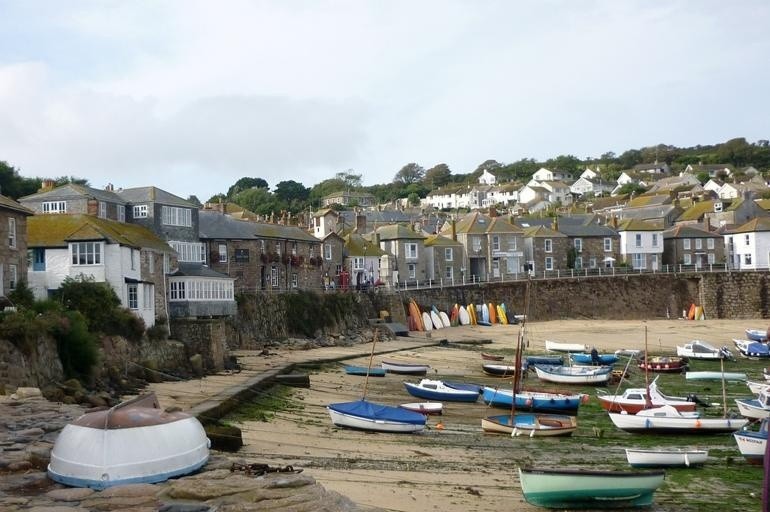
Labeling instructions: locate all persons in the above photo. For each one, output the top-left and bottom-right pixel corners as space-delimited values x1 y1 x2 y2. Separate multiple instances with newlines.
323 279 329 292
330 278 336 291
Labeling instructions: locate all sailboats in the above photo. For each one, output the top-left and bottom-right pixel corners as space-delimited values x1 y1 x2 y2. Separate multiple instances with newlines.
607 324 751 435
325 326 426 433
481 268 589 438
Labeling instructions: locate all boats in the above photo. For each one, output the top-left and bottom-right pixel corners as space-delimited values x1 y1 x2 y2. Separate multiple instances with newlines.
518 467 665 508
480 327 770 393
343 365 386 377
402 377 480 403
399 402 443 416
625 446 708 466
734 390 770 466
380 360 430 375
